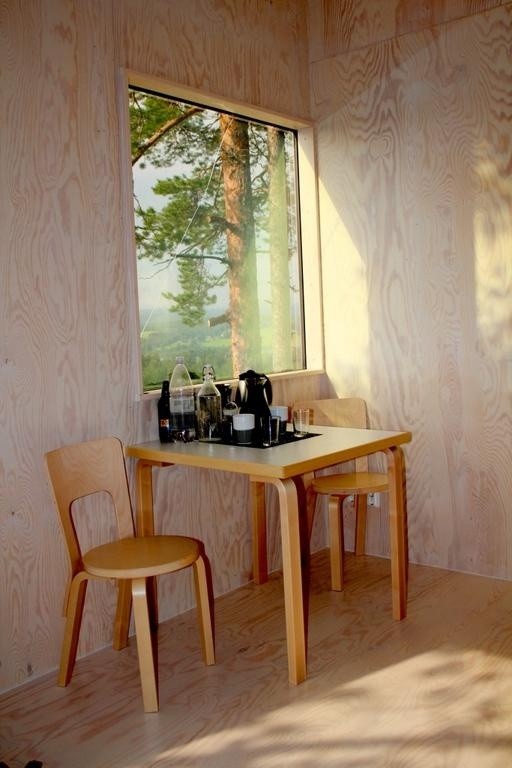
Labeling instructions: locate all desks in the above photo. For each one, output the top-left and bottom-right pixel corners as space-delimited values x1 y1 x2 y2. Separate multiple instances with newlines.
123 419 412 688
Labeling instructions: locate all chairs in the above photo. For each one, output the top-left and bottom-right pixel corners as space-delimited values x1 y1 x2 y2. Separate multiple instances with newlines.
42 436 217 715
290 396 391 592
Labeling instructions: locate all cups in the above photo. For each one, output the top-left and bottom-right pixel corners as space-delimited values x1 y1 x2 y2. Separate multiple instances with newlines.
260 415 281 447
270 406 289 435
232 413 256 445
290 400 311 438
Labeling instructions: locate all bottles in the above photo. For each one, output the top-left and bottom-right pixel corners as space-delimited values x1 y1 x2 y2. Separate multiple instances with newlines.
167 355 197 445
195 364 223 443
156 379 172 444
